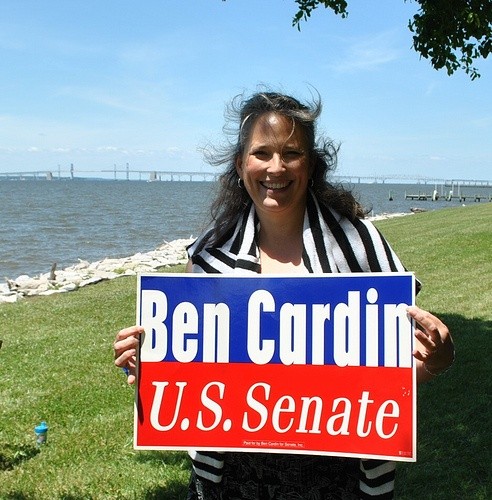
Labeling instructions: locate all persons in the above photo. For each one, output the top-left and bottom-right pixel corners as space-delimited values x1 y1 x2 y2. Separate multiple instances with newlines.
114 80 454 500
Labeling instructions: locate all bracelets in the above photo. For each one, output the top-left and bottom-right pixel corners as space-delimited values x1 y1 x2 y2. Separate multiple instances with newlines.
423 352 457 378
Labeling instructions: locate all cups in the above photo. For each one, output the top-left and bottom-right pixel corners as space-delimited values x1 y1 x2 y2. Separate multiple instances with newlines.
34 422 48 446
